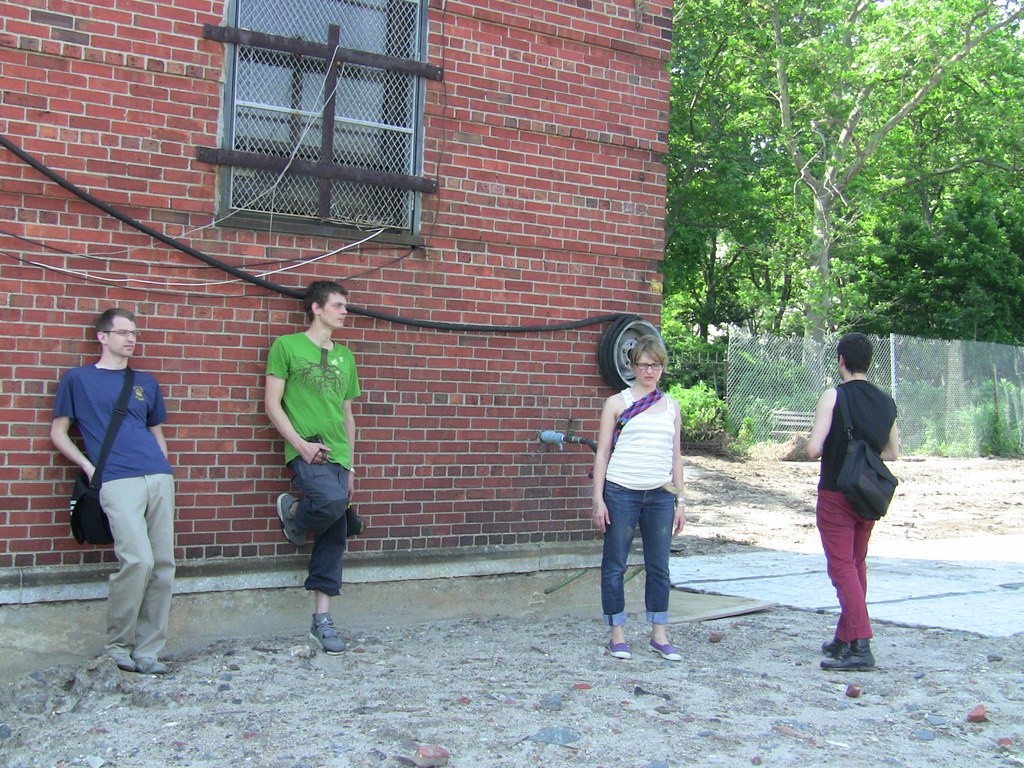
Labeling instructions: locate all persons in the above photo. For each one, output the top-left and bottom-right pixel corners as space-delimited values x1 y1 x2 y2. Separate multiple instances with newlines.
805 334 900 670
264 282 361 656
50 307 177 674
591 336 686 661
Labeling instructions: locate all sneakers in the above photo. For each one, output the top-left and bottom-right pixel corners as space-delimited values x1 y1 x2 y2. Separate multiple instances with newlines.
277 493 307 547
136 657 167 674
309 613 346 655
109 653 135 671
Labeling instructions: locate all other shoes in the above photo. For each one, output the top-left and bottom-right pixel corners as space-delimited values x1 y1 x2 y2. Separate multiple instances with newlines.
650 638 681 661
609 639 631 659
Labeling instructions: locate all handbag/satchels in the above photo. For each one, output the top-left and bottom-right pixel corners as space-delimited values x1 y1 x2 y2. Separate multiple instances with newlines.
838 439 898 521
70 473 114 546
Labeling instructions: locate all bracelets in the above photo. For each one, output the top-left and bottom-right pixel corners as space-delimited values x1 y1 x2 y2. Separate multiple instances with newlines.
349 468 357 474
677 505 685 508
677 498 685 501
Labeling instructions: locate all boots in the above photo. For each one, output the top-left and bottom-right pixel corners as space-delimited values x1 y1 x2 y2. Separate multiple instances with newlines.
823 637 849 659
820 639 876 670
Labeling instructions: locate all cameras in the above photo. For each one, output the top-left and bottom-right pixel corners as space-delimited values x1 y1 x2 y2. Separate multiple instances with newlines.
306 434 327 455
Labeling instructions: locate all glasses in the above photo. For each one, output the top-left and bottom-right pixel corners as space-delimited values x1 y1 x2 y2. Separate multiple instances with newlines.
636 362 661 370
104 329 140 337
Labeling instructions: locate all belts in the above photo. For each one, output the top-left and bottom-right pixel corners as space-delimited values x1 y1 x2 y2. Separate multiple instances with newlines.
664 483 677 494
